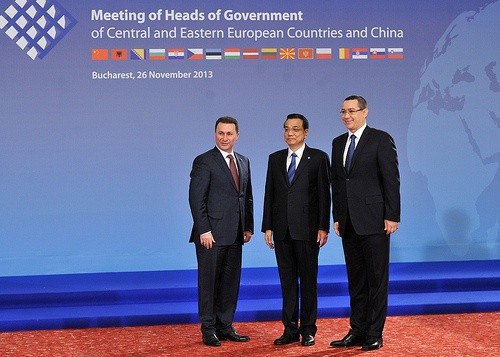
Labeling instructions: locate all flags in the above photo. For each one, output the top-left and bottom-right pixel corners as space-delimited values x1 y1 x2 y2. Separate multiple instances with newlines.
316 47 332 59
168 48 184 60
206 48 222 60
148 48 165 61
130 48 146 60
92 49 109 61
224 48 241 60
370 47 386 59
111 49 129 61
333 47 350 61
352 48 368 60
279 48 296 60
388 47 404 59
243 48 258 60
187 48 204 60
261 48 277 59
298 48 314 59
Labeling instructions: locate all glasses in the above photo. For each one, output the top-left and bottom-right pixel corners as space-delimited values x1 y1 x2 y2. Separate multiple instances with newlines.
340 107 366 116
283 128 305 132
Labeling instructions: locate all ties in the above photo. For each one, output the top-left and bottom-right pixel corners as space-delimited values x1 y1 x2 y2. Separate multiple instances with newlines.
287 153 297 184
345 135 356 176
228 155 239 191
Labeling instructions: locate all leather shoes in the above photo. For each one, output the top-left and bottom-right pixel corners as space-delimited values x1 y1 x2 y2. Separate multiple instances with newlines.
301 334 316 345
330 334 366 347
361 337 383 350
273 332 299 345
202 333 221 346
216 331 250 342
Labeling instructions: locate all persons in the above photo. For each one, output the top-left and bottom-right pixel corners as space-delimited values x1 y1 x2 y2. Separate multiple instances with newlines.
188 117 254 347
261 114 331 346
330 95 401 351
